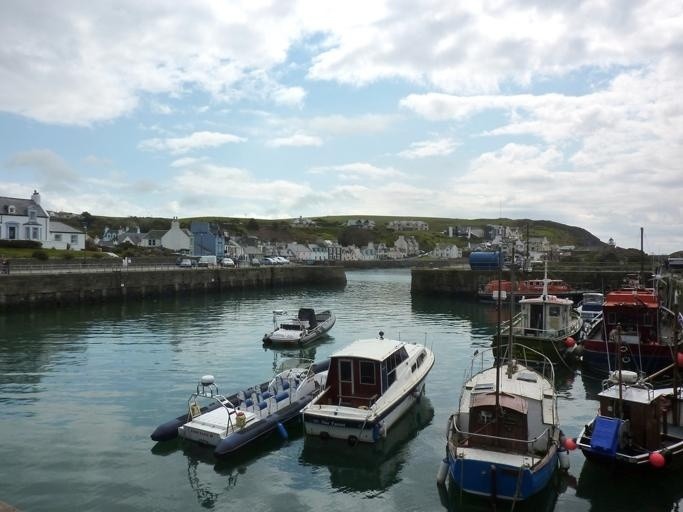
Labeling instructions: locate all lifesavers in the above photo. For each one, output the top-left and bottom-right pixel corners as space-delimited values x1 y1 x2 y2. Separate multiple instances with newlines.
622 357 630 363
620 346 628 352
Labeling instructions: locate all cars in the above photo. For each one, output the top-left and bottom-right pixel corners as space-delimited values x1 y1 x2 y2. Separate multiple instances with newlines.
250 257 289 266
198 257 209 267
220 258 235 267
180 259 192 267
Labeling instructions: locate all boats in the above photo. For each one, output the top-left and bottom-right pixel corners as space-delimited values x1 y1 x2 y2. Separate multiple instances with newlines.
490 258 584 367
575 293 606 321
300 331 435 448
151 358 331 459
446 238 561 501
263 308 336 348
581 228 683 380
575 312 683 471
478 277 601 304
